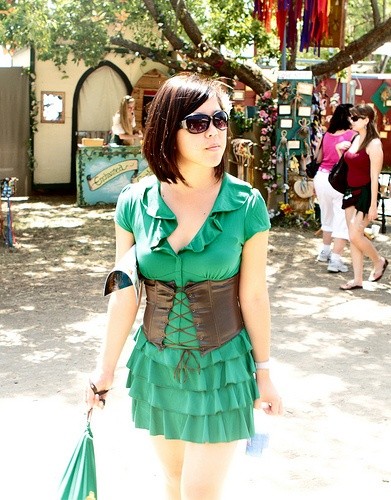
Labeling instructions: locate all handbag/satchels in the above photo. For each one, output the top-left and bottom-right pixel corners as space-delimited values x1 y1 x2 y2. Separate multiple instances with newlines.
328 134 358 194
55 421 97 500
305 132 328 178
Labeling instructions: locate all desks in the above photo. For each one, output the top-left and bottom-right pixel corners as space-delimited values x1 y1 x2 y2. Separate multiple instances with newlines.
74 142 147 208
0 195 31 248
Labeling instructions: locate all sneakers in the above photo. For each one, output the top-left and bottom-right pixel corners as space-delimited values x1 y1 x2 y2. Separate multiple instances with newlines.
318 249 332 262
327 259 349 273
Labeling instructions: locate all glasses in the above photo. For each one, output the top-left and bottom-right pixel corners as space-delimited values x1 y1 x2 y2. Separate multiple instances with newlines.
351 115 365 122
178 110 229 134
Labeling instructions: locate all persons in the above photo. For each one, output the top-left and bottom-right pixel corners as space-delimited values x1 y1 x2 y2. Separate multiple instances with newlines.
311 102 355 271
88 75 281 500
337 105 388 288
110 97 144 144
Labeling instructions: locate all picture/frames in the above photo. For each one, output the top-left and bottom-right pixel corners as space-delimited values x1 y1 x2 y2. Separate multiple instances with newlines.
40 91 66 124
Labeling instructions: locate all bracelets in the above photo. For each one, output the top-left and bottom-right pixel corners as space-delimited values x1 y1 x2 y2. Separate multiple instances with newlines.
253 357 274 370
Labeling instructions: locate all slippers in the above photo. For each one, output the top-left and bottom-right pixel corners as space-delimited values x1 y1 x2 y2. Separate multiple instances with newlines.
368 257 389 282
339 282 363 290
